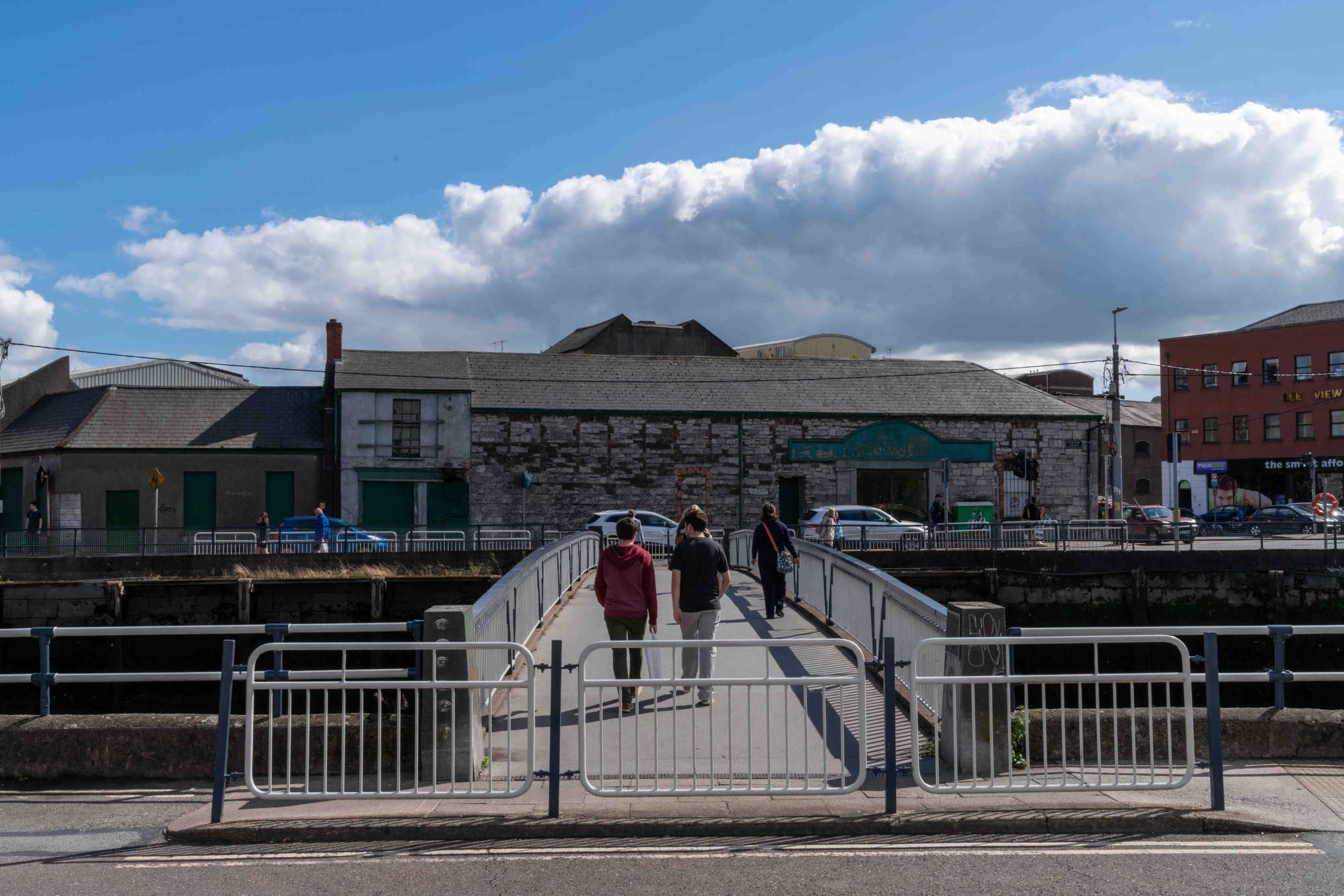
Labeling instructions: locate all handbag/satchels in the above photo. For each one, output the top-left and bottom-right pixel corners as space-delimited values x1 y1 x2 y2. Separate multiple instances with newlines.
815 517 824 534
644 632 662 690
776 552 793 574
319 540 328 553
674 534 684 545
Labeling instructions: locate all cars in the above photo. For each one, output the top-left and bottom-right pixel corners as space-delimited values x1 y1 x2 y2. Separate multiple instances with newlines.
1167 507 1208 537
272 515 389 555
1098 495 1133 520
1243 501 1344 537
1193 503 1257 535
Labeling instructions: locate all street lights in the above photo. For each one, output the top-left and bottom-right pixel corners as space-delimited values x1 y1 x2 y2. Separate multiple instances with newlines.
1110 306 1131 545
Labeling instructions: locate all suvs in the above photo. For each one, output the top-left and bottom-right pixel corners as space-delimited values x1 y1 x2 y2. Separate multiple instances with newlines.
1120 503 1199 545
583 508 714 558
797 504 933 553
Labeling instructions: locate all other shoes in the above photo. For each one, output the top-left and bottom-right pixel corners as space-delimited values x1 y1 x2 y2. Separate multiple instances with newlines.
775 609 784 616
682 685 692 692
766 612 775 619
27 552 38 557
630 686 643 698
1024 534 1029 543
1036 541 1043 545
702 697 715 704
622 703 634 712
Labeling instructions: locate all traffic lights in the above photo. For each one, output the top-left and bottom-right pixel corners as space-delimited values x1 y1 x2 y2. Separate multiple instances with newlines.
1013 448 1027 480
1026 457 1039 481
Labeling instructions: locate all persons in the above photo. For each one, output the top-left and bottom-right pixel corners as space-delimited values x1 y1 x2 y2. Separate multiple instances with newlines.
627 509 646 547
930 494 958 533
254 512 270 554
819 508 843 552
595 518 658 711
878 505 887 522
1150 510 1160 518
968 511 986 529
750 503 801 620
1208 474 1272 509
667 505 731 705
1022 497 1044 545
313 508 331 553
24 502 43 556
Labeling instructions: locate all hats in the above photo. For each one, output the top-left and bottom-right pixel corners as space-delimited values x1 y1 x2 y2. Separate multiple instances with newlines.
935 494 943 498
